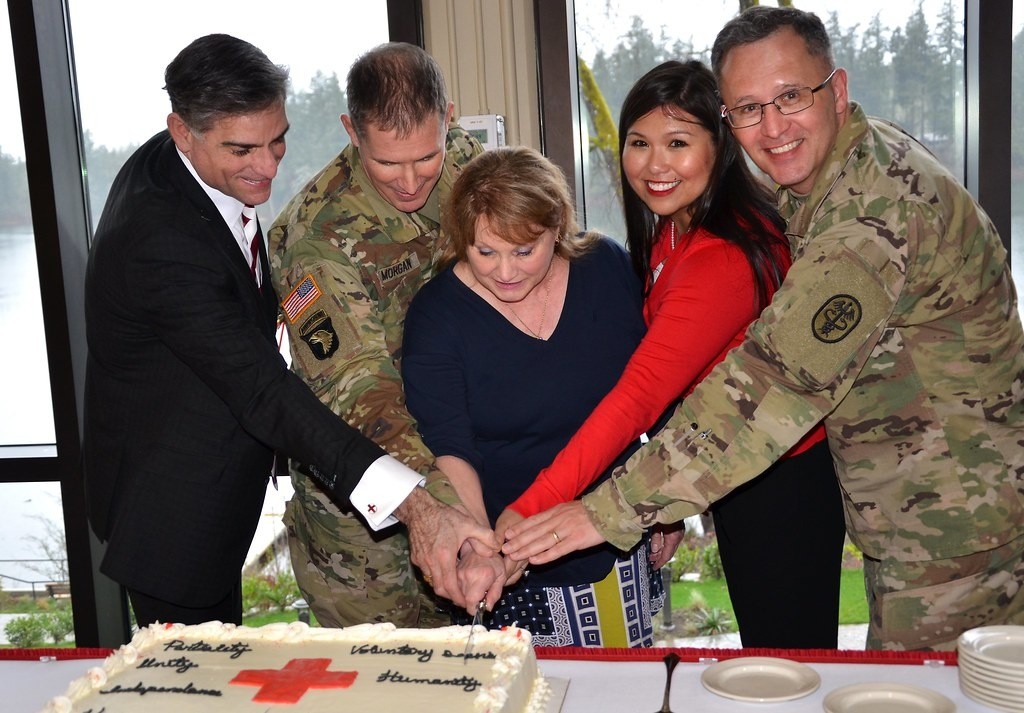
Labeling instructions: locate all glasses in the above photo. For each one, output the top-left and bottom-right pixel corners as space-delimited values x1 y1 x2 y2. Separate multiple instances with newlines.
721 68 838 130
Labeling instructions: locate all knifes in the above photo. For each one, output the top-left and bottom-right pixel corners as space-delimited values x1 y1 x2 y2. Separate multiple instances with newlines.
463 600 485 666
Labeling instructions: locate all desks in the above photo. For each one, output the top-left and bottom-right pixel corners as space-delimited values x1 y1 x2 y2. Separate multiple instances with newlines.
0 645 991 713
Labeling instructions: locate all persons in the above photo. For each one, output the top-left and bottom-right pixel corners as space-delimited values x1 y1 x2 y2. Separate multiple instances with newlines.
502 6 1024 654
494 60 847 650
78 33 500 629
403 148 686 649
265 43 486 629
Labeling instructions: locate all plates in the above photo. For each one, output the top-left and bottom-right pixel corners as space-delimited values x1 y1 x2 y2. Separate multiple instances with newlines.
957 626 1024 713
823 682 957 713
700 656 821 703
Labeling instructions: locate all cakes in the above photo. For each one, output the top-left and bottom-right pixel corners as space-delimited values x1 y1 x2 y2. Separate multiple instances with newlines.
43 620 547 713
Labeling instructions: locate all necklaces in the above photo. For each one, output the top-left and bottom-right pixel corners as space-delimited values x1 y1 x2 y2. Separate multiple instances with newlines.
503 262 553 339
672 220 692 249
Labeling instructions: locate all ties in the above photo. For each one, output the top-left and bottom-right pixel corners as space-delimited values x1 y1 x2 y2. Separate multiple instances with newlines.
241 203 292 371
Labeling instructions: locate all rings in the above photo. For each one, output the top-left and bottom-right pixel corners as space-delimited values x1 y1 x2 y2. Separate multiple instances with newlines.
549 529 561 543
520 566 530 576
422 574 432 581
